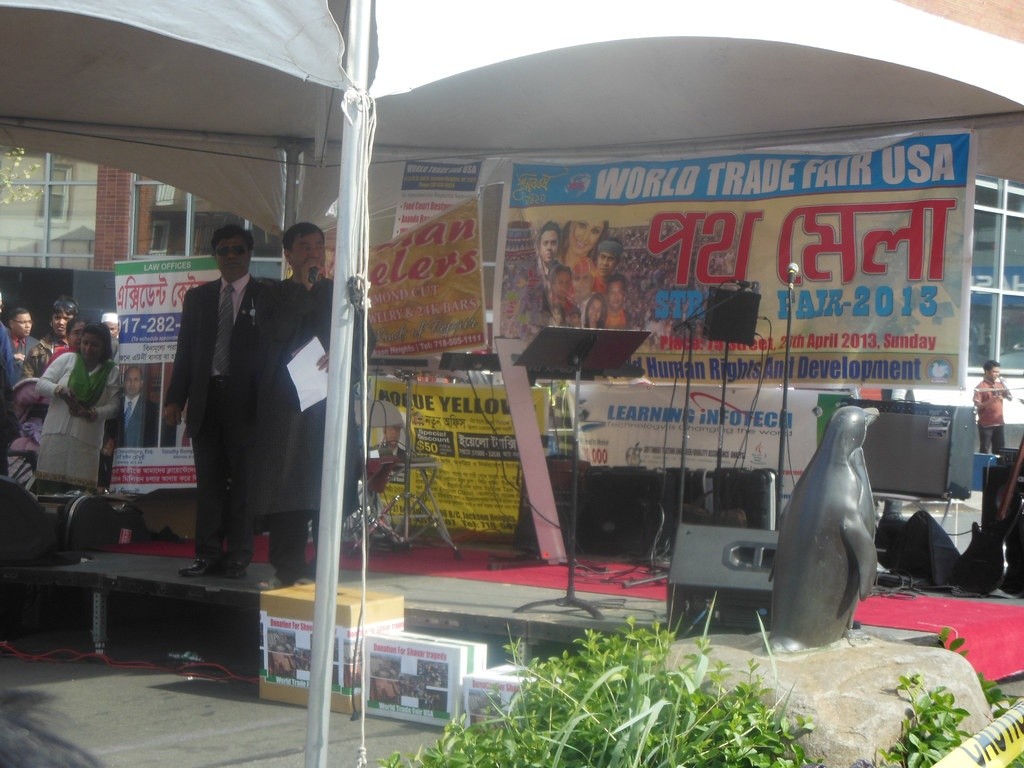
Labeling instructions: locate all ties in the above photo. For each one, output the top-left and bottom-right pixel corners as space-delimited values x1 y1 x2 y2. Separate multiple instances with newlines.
212 284 234 376
16 340 24 368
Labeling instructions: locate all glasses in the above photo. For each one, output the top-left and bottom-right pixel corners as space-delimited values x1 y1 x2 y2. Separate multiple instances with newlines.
53 300 77 308
216 243 249 257
14 320 33 324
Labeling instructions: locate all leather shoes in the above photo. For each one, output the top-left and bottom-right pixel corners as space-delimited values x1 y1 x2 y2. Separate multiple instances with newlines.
222 562 246 579
177 556 221 577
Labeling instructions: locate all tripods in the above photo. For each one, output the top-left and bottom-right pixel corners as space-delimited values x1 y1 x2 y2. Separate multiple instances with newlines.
347 372 463 564
510 323 652 620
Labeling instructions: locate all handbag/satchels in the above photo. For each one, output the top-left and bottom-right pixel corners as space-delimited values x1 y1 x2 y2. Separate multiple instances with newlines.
57 491 150 551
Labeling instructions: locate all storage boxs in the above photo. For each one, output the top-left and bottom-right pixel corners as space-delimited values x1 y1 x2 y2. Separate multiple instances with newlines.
259 582 579 728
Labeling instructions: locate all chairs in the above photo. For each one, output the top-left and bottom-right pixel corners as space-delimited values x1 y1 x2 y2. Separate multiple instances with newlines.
9 492 144 623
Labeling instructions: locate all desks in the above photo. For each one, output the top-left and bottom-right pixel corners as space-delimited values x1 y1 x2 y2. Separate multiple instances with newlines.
873 493 964 556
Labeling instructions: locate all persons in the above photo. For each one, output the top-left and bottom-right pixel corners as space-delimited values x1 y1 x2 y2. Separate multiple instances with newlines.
162 225 268 577
379 426 405 455
874 388 916 520
502 219 738 328
252 222 376 584
118 368 153 449
972 360 1012 454
0 293 119 491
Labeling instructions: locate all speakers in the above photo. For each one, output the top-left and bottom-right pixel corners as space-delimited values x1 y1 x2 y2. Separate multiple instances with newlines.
0 476 150 566
667 524 781 641
702 287 760 347
514 460 582 557
840 398 977 501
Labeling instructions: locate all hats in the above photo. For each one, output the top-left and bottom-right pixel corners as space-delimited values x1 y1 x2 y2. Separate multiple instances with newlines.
100 313 119 323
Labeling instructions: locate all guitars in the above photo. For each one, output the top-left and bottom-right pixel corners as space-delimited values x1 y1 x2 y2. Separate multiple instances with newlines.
949 436 1024 594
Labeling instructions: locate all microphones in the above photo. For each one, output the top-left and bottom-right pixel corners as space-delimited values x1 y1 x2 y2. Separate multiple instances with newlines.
786 262 799 290
307 266 319 283
730 278 760 291
